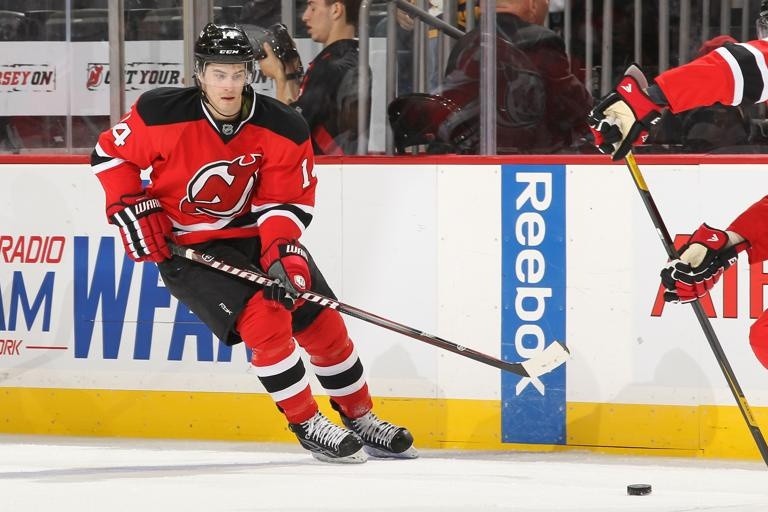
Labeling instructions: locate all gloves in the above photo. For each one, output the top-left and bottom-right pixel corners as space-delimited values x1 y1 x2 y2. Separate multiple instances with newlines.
585 61 671 161
109 193 179 263
660 222 751 305
258 237 312 312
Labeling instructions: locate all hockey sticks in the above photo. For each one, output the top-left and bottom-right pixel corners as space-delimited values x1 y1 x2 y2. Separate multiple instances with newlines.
165 239 570 377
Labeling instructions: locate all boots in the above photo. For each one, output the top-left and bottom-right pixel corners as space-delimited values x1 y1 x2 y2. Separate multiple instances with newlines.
329 397 415 454
275 401 365 459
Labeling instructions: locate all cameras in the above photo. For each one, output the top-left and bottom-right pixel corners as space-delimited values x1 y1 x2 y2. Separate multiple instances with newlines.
248 23 299 62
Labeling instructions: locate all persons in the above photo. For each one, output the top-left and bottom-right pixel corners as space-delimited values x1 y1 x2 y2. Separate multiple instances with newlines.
425 1 601 155
396 0 483 94
87 20 415 457
586 35 768 303
257 0 372 155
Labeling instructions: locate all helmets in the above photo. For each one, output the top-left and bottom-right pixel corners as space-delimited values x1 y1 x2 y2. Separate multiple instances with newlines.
193 22 255 89
756 0 768 39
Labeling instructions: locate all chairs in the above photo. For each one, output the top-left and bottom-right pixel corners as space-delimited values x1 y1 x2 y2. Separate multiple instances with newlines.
384 91 480 156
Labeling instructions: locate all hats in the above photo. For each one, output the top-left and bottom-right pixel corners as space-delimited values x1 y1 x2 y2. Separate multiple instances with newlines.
696 34 740 56
